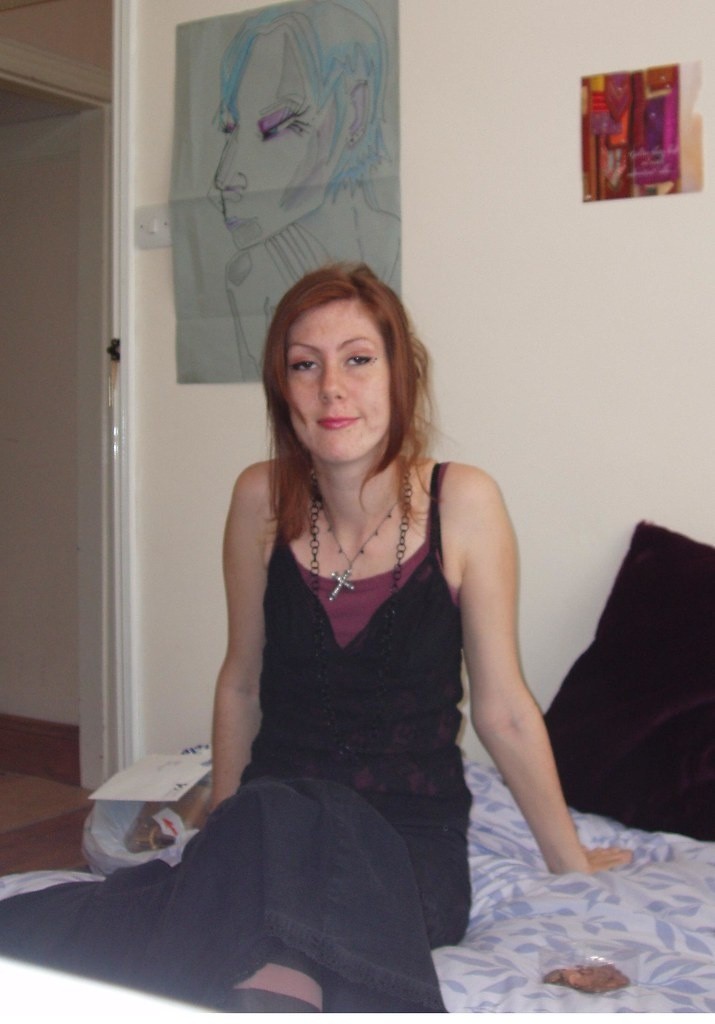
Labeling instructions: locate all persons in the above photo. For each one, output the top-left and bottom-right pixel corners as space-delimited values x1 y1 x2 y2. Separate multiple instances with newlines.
0 263 633 1013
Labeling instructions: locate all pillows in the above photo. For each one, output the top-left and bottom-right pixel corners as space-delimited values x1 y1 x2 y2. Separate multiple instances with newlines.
546 523 715 843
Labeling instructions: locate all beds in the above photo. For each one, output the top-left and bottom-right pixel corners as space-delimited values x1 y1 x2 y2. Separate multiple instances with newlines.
0 762 715 1014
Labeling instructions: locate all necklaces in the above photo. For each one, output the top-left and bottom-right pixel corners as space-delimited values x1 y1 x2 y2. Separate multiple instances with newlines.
322 504 398 602
